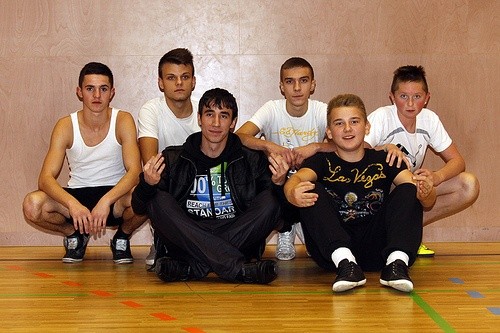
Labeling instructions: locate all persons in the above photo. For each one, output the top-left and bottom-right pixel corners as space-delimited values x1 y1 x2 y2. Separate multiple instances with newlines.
234 58 373 261
284 94 437 292
132 48 289 286
23 62 148 264
364 65 480 258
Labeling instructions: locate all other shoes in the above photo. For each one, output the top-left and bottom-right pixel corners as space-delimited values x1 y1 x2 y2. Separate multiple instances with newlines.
145 244 156 265
416 242 435 255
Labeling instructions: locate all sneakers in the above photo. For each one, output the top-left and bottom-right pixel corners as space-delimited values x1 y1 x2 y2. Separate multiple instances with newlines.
238 259 279 283
379 259 414 292
110 228 134 264
332 258 366 292
296 222 312 256
62 229 90 263
276 225 296 261
155 257 193 282
